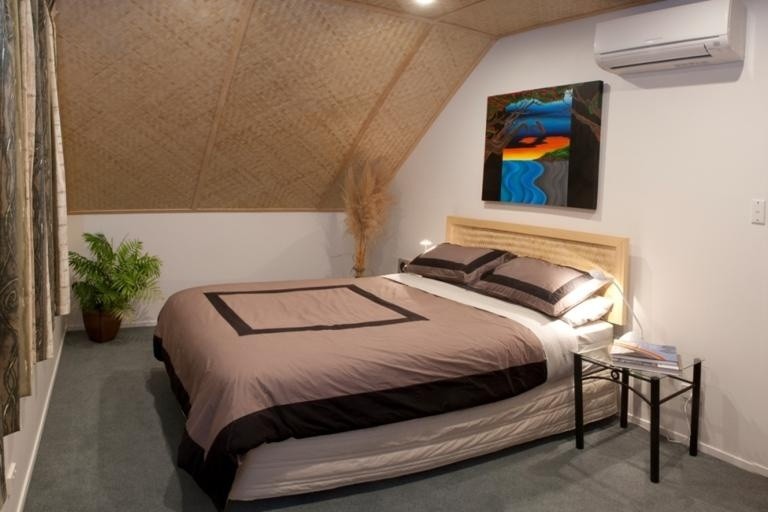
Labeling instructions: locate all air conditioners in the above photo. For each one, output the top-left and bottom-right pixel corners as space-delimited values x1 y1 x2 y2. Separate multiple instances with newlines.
592 0 747 75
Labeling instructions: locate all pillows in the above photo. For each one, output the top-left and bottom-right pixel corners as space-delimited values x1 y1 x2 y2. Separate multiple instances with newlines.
464 256 609 317
402 242 518 283
559 293 614 328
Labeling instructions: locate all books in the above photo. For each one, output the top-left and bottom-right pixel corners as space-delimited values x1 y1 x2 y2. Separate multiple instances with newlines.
609 338 680 372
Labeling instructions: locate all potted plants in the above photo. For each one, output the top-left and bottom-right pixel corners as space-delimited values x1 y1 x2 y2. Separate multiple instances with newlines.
66 232 165 344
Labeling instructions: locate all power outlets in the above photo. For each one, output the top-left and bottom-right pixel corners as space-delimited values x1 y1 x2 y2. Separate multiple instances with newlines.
751 199 766 225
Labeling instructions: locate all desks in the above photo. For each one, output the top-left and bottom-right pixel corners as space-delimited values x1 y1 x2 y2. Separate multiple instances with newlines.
568 336 705 484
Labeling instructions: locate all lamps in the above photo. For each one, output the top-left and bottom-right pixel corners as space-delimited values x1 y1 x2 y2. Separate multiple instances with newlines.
591 269 644 355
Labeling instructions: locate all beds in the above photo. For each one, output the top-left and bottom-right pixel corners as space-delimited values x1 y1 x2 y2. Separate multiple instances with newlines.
170 216 631 502
